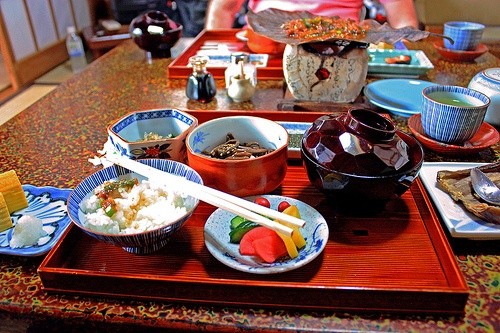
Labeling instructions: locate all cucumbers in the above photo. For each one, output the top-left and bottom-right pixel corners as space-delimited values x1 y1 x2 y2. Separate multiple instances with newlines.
229 211 272 243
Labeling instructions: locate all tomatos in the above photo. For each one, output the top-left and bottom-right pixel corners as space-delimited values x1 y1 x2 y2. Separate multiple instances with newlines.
278 201 291 212
254 197 271 207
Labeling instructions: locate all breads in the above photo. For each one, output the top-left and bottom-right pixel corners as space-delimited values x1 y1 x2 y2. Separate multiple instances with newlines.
0 169 29 232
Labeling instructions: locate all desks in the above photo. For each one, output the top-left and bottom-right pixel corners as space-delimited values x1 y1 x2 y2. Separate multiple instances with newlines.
82 24 132 61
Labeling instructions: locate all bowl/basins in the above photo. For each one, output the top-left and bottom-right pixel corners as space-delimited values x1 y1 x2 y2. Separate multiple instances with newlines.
65 159 203 256
186 116 289 198
467 67 500 134
129 24 182 59
305 107 414 176
299 130 424 203
245 29 285 56
129 11 178 32
106 108 198 166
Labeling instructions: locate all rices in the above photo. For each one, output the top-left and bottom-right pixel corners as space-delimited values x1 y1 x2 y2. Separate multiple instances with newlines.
10 214 47 248
85 177 187 234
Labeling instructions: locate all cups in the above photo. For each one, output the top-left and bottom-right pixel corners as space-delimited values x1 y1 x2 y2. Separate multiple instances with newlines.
443 21 486 50
421 84 490 144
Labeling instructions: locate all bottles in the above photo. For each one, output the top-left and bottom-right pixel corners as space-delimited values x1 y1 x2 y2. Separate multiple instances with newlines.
66 26 88 73
184 52 258 102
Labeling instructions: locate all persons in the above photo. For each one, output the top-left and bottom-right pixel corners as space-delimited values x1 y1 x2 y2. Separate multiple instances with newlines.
204 0 420 34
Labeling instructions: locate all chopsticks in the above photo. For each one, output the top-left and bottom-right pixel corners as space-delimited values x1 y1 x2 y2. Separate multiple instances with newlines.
106 152 306 238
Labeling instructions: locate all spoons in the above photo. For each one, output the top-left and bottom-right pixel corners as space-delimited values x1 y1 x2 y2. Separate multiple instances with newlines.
471 167 500 206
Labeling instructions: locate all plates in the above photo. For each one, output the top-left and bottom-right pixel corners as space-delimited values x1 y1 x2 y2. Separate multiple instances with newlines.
367 49 435 74
1 184 72 257
273 120 312 158
203 195 331 275
186 54 268 68
363 80 440 117
433 41 488 61
417 161 500 239
394 40 408 49
236 30 248 41
408 114 500 154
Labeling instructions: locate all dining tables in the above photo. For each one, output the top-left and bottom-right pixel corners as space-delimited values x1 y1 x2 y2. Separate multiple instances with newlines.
0 32 500 333
